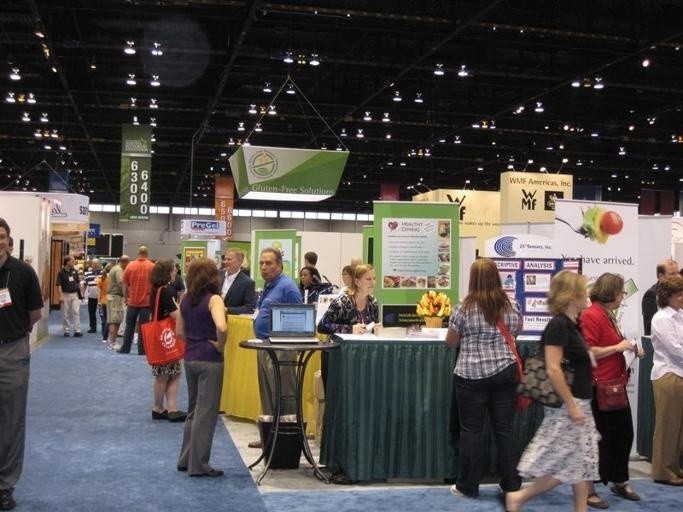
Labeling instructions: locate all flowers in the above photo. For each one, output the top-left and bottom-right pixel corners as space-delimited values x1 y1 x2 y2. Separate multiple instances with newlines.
415 290 451 318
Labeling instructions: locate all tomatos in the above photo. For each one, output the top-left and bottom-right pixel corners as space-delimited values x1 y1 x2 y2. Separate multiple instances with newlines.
601 211 623 235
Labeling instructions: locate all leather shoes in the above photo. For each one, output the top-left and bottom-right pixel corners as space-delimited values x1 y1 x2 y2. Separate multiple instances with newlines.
150 409 188 422
249 441 261 448
654 477 682 486
206 469 224 476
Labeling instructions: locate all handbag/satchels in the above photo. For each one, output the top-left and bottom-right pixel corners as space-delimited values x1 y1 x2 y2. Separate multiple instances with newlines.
518 355 575 412
141 315 188 366
598 377 626 409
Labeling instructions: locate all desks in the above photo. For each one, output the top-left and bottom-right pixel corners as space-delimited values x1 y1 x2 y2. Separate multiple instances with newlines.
216 312 325 437
321 331 542 484
238 338 340 486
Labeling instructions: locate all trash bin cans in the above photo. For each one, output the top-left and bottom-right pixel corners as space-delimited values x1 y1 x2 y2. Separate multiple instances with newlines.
258 420 307 469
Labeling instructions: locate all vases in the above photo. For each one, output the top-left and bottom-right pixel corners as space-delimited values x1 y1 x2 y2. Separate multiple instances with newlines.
424 316 444 328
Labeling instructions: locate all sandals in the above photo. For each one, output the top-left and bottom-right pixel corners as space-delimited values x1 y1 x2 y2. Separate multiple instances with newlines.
451 483 464 497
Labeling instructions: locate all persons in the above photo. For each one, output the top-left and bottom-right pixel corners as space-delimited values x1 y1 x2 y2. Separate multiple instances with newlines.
503 269 602 512
650 273 683 485
578 272 646 509
175 256 230 476
445 256 524 500
641 260 683 336
80 257 104 334
55 255 83 337
0 218 44 512
217 244 383 448
99 246 190 423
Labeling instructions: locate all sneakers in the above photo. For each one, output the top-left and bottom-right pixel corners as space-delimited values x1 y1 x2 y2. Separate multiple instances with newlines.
610 483 641 500
587 493 609 509
1 488 16 509
64 329 123 350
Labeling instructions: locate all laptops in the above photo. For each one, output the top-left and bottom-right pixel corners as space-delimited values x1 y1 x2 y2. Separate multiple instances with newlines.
268 303 319 344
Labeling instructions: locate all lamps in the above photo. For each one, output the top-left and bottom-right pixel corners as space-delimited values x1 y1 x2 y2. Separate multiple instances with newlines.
227 77 349 202
